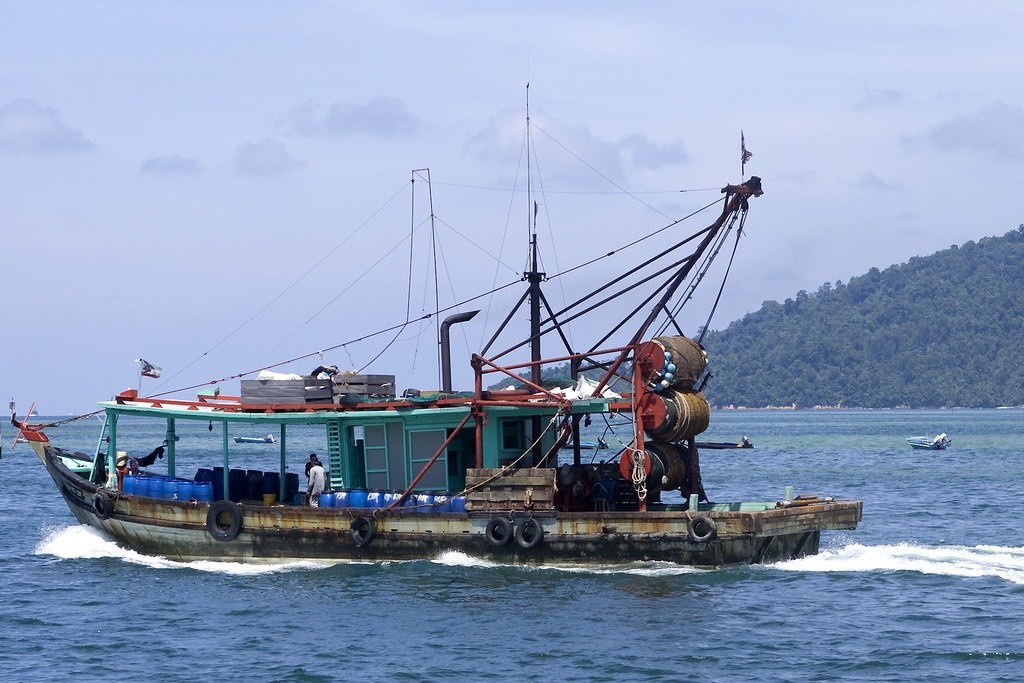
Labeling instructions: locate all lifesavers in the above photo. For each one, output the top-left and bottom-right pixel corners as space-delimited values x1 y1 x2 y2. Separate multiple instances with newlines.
91 492 113 520
515 518 544 549
350 517 376 545
486 516 513 547
206 500 243 543
688 515 717 543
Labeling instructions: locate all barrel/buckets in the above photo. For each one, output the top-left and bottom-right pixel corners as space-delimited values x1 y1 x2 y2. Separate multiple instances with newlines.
349 487 367 508
417 491 433 513
333 488 349 510
120 468 304 507
366 488 385 508
318 491 336 509
383 490 400 510
399 491 416 512
450 491 467 513
433 490 451 512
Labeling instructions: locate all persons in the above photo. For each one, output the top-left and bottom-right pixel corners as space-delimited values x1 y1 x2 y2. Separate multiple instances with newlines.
304 453 325 506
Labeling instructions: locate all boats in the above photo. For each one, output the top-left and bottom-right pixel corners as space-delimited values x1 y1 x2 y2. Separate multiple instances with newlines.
233 434 277 444
561 436 609 449
14 438 28 443
906 433 951 450
695 436 754 449
11 176 864 569
28 411 39 417
